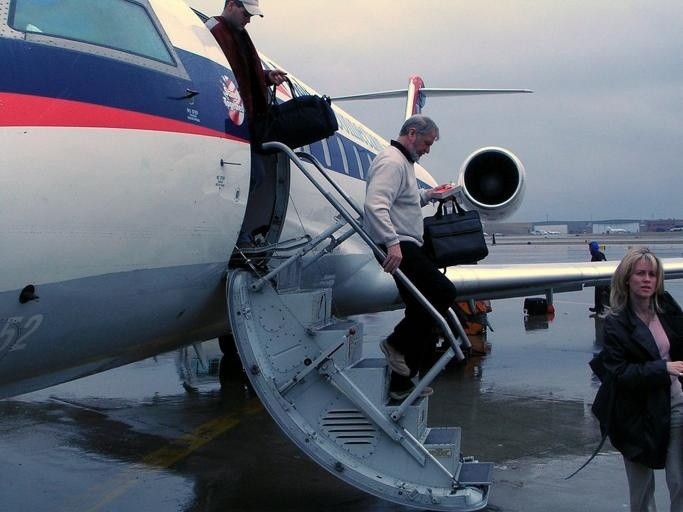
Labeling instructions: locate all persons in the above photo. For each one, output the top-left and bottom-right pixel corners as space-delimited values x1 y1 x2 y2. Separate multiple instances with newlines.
363 113 458 401
588 248 683 512
587 240 607 312
204 0 288 248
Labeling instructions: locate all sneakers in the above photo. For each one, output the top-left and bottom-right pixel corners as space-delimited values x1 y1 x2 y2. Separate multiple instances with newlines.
389 384 434 400
589 308 596 312
231 241 266 258
379 339 411 377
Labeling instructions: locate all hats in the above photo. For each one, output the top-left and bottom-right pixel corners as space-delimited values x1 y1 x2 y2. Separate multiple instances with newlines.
238 0 264 18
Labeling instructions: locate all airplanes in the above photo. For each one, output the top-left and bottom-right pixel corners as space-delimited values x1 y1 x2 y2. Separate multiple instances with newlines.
0 0 683 512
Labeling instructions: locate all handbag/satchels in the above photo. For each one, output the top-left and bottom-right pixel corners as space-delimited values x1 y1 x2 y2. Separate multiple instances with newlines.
423 195 489 275
250 75 338 155
